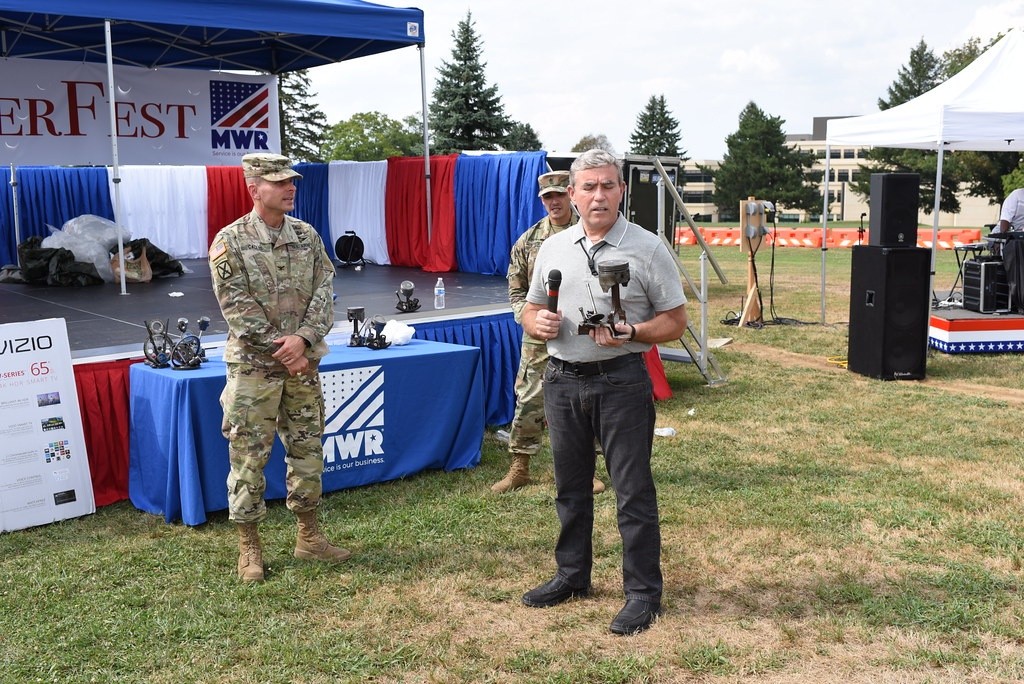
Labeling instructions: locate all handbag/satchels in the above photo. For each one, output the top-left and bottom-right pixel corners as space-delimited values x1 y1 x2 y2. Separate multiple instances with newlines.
111 246 152 284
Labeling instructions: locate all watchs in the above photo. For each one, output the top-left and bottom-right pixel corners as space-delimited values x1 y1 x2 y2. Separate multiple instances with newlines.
625 324 638 345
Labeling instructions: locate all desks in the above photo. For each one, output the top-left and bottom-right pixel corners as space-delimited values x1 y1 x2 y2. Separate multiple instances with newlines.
128 335 486 526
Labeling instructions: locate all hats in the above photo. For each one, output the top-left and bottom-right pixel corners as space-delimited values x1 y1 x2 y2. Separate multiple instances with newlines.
538 170 570 197
241 153 303 182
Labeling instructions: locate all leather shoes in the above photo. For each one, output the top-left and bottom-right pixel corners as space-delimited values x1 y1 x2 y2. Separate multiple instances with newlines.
522 578 590 607
609 599 663 634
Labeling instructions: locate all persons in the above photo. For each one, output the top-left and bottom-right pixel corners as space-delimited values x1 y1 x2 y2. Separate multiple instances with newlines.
488 170 606 495
990 187 1024 260
518 147 689 637
209 152 353 585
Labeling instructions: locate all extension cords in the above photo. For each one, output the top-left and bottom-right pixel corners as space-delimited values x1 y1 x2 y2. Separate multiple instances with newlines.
939 300 963 308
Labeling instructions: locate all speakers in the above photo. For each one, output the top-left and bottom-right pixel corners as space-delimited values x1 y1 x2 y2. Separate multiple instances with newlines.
868 172 921 247
847 245 933 379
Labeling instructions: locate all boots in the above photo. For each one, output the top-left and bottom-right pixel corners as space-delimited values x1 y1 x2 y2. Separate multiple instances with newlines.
490 454 529 494
592 476 606 493
235 523 264 583
293 508 351 563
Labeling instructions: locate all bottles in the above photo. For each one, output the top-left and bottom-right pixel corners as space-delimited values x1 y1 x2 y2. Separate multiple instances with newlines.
434 277 445 310
496 429 509 443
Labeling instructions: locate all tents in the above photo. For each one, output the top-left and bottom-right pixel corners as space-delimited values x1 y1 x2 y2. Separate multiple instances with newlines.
820 20 1024 330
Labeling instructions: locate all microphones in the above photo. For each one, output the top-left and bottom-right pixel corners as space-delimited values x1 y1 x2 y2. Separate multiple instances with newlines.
547 270 562 315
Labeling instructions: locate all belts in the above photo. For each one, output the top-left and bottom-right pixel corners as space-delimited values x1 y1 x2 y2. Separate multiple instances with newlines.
550 353 642 375
